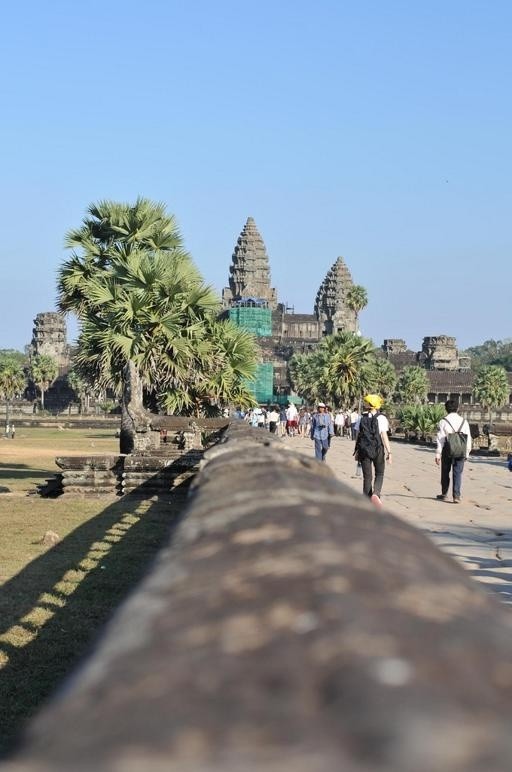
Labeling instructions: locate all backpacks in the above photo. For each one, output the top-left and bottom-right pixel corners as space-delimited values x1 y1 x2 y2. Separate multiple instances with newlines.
358 413 387 465
442 417 468 459
345 416 351 426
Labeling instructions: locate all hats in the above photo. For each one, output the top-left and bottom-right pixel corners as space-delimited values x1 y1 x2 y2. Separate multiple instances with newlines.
317 403 325 408
363 395 382 409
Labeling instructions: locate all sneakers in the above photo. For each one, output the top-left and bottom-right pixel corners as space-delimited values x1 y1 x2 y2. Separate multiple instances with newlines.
453 497 462 503
437 495 449 501
371 495 381 504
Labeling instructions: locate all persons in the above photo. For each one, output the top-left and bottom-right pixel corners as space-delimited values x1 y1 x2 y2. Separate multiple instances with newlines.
351 393 393 511
434 399 472 503
308 402 331 464
232 400 362 442
11 424 15 439
5 424 9 439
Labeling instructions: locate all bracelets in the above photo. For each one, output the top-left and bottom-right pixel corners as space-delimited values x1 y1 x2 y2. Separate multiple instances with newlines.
386 452 392 454
